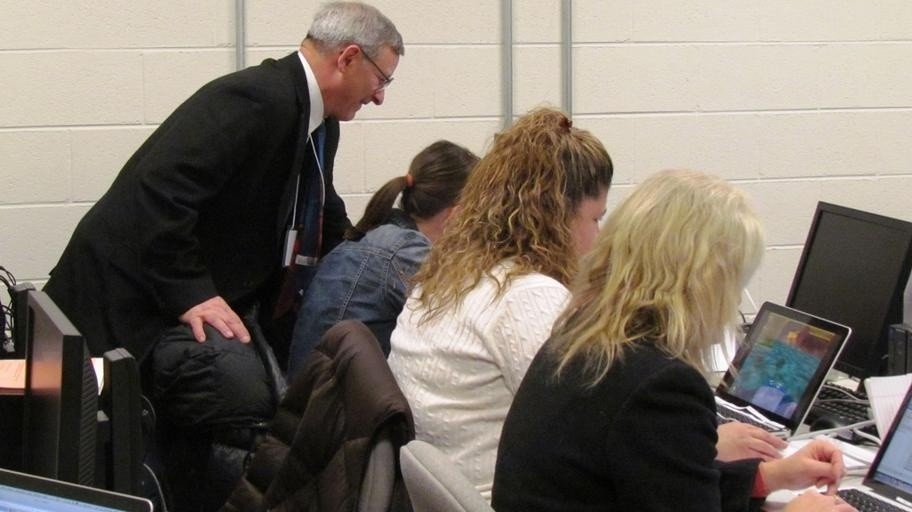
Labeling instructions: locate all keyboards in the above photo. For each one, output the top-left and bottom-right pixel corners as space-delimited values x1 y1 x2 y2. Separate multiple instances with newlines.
804 378 880 443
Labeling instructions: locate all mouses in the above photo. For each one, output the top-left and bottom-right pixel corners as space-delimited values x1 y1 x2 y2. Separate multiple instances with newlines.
809 415 853 442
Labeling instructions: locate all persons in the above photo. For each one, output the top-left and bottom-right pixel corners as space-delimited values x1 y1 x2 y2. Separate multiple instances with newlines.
385 104 789 507
489 167 860 512
41 0 406 363
285 139 484 394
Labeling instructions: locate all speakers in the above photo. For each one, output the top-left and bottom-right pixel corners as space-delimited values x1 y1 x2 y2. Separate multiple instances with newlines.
887 322 911 376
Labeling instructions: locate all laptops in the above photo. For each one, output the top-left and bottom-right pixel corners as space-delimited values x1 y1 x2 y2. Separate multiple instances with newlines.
713 302 853 441
0 468 154 512
761 383 912 512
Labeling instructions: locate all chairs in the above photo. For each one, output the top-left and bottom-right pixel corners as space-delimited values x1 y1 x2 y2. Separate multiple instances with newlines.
2 268 494 510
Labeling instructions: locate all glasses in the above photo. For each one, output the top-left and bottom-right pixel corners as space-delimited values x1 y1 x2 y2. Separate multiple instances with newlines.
343 45 394 93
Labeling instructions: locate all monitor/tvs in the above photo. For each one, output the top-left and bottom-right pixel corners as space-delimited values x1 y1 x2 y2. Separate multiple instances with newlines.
785 200 912 400
24 291 142 511
